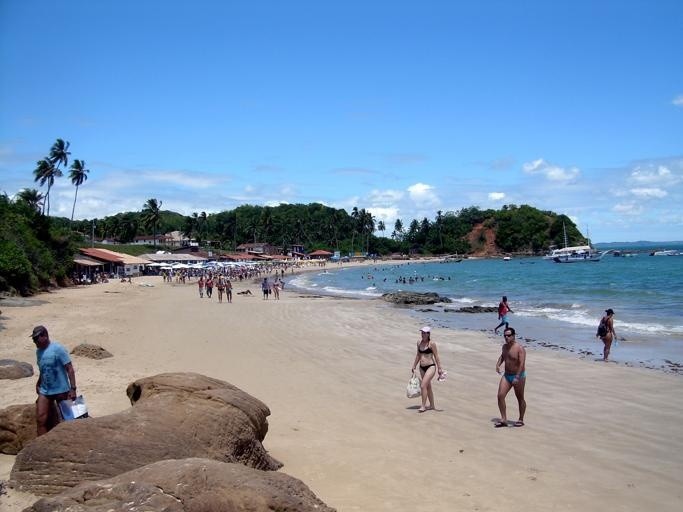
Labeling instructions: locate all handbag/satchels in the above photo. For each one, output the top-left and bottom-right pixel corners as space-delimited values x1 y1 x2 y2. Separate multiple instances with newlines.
406 377 422 397
60 396 88 419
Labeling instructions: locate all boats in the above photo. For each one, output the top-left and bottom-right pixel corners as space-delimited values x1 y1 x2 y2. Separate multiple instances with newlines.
621 253 639 257
439 253 462 263
504 253 512 260
650 250 683 256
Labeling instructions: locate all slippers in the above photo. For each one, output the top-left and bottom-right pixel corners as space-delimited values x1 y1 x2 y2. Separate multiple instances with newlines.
495 422 508 427
514 422 524 426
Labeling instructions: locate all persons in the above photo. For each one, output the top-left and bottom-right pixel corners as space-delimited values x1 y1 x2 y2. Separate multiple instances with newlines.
27 324 77 438
595 308 619 362
493 327 527 428
396 275 451 285
493 295 515 334
74 257 328 302
409 325 443 412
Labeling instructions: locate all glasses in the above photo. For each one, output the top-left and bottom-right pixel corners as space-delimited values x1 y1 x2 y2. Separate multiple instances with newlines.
504 334 513 337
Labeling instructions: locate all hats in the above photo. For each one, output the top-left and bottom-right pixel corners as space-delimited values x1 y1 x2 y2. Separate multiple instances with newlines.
419 327 430 333
30 326 47 336
607 309 615 314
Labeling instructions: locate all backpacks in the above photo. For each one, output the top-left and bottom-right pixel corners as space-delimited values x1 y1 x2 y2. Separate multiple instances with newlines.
598 317 610 337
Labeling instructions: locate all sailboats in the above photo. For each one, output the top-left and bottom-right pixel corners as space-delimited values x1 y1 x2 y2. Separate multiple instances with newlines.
540 220 613 262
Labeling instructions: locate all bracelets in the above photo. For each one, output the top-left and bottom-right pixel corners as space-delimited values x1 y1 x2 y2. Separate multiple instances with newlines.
69 385 77 391
